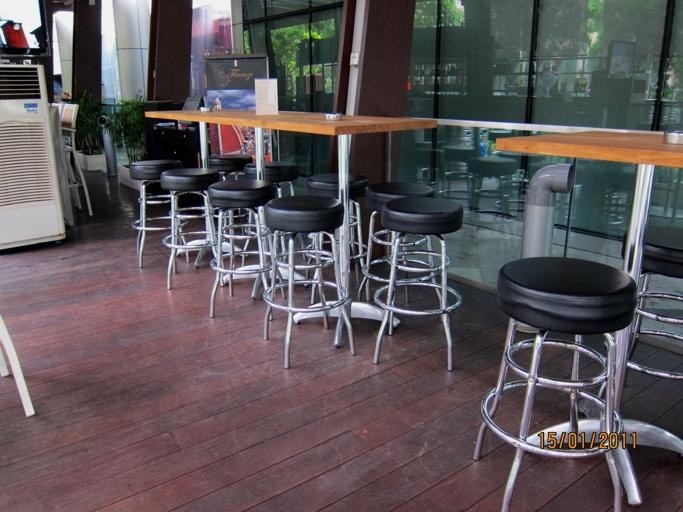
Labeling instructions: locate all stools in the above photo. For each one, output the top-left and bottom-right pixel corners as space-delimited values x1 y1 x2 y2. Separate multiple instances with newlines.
161 168 225 290
472 256 638 512
241 161 307 266
413 143 631 228
598 225 683 409
208 152 253 182
356 182 449 323
372 197 463 372
130 160 190 269
305 172 370 304
208 177 287 321
263 193 356 369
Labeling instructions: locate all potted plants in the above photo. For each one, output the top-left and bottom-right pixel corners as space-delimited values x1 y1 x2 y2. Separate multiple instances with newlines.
75 90 107 172
107 97 160 192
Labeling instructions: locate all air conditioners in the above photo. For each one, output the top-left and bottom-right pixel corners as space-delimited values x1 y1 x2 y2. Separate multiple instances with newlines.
0 65 67 253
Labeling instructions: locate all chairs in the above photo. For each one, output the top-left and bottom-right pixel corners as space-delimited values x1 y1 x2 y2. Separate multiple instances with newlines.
58 103 94 217
